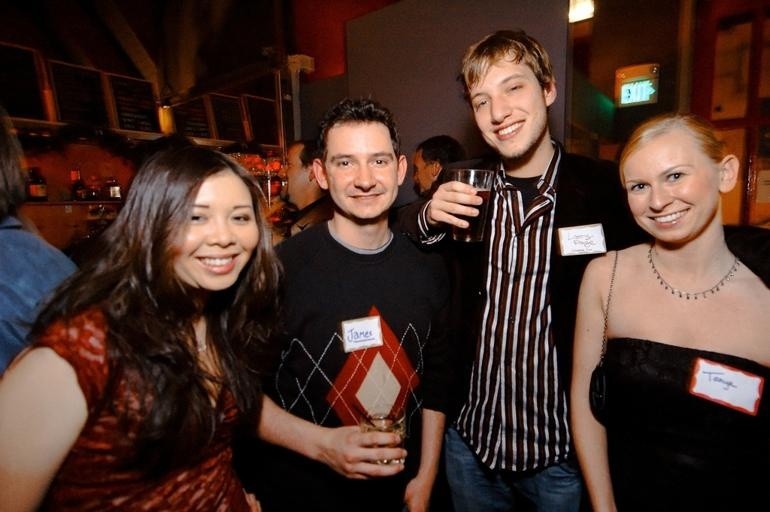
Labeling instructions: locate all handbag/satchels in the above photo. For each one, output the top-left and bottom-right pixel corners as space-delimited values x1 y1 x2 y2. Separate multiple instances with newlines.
589 360 607 429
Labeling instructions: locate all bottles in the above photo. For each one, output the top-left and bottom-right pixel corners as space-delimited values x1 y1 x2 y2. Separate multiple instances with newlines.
23 161 128 204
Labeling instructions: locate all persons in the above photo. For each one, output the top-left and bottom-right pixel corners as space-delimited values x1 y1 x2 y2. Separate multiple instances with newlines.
386 24 655 512
410 135 466 196
269 135 333 247
2 144 289 512
228 94 461 512
570 110 770 512
0 126 82 380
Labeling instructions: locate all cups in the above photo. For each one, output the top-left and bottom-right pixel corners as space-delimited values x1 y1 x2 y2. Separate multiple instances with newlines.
359 403 409 472
449 167 495 243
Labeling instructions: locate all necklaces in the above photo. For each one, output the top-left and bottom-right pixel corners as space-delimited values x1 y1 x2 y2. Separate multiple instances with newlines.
648 243 741 300
193 345 206 353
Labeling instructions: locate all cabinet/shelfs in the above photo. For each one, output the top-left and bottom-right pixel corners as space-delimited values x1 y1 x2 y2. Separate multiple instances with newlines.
21 201 123 263
